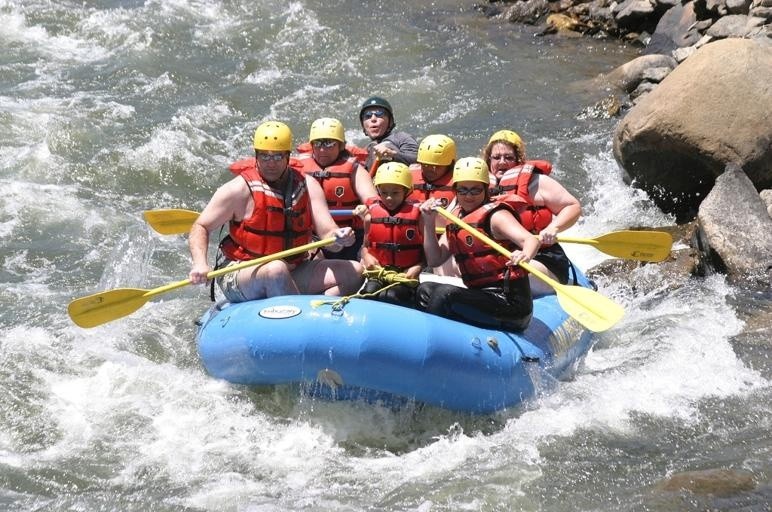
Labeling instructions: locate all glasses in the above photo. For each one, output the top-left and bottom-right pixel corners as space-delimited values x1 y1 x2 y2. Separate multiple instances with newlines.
312 140 337 150
455 186 485 196
488 154 517 163
257 152 288 162
361 111 388 121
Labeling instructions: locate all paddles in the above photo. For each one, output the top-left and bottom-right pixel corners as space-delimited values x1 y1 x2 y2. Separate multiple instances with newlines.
67 236 339 328
145 208 362 234
430 204 623 332
436 228 671 263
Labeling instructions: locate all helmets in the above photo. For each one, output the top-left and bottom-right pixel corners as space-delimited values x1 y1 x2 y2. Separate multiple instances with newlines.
416 133 458 167
308 117 346 145
487 129 526 149
359 96 395 128
253 120 293 153
452 156 490 185
373 161 413 192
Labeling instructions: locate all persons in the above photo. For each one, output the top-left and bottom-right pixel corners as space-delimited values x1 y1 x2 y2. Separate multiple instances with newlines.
418 154 535 332
294 115 384 264
482 127 583 300
187 120 366 303
346 95 420 166
355 161 436 307
407 132 498 242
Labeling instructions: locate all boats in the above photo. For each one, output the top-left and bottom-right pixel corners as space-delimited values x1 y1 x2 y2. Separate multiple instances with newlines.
190 253 608 415
